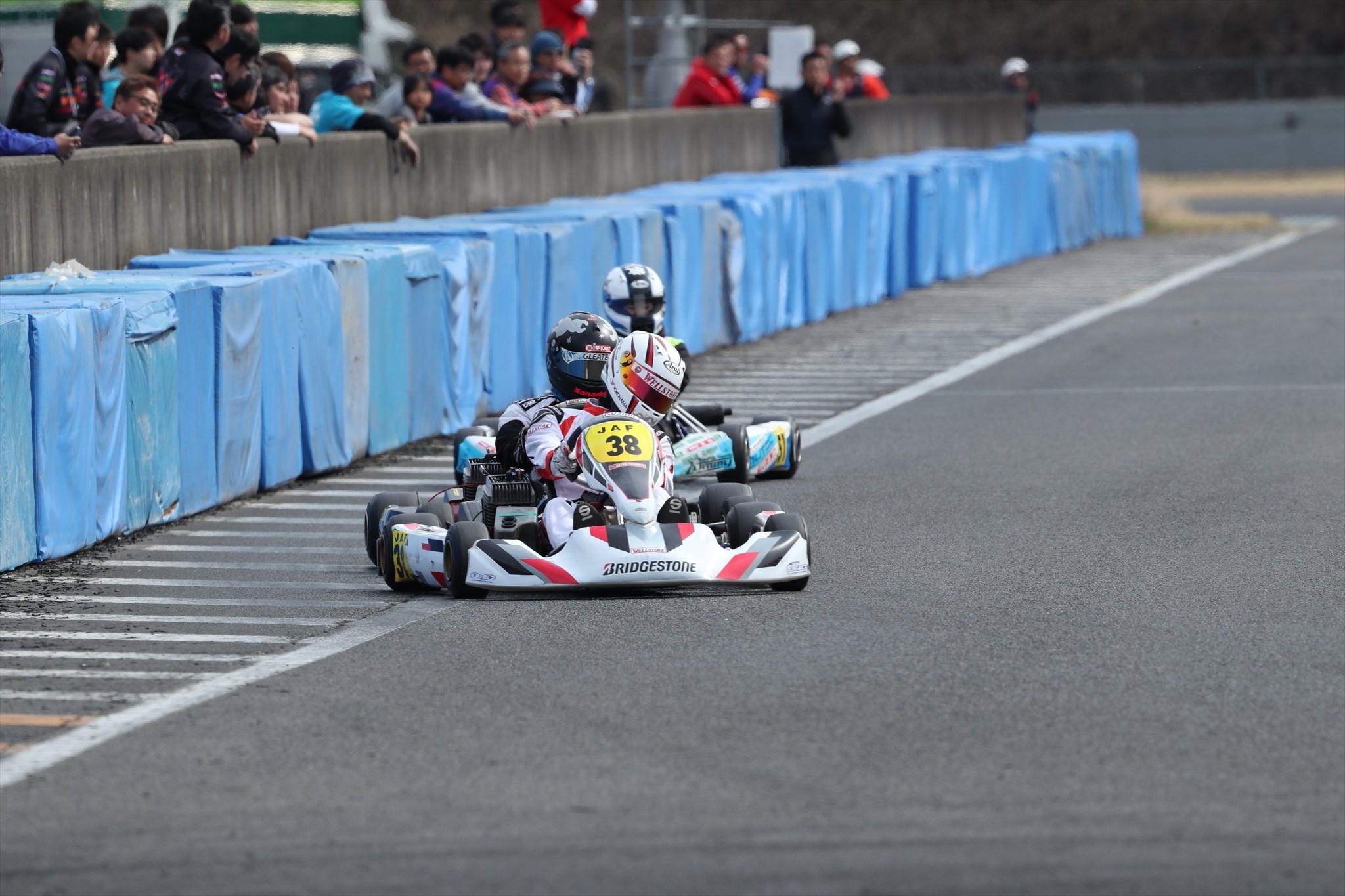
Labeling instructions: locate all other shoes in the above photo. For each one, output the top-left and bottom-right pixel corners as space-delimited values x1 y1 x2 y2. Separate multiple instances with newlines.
574 502 606 531
657 497 691 525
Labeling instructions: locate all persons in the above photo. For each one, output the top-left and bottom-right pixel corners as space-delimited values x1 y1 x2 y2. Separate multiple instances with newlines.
0 0 614 169
522 331 685 551
672 27 889 111
777 53 854 172
494 309 619 473
599 262 690 400
1001 56 1042 141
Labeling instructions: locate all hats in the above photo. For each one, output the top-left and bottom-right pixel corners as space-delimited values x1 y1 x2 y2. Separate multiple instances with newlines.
532 31 562 58
332 59 376 93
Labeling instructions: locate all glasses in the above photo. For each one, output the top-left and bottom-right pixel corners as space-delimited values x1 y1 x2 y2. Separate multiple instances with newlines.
546 51 564 56
133 95 159 110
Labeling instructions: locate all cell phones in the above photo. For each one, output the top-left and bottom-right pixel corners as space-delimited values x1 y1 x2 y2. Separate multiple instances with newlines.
68 126 80 137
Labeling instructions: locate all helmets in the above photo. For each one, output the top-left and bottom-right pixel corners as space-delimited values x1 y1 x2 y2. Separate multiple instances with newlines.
1000 57 1031 82
601 329 687 427
832 40 859 64
545 311 619 399
603 262 667 336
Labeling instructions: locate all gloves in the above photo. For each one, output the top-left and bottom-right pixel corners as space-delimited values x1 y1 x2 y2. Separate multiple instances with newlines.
550 440 579 475
